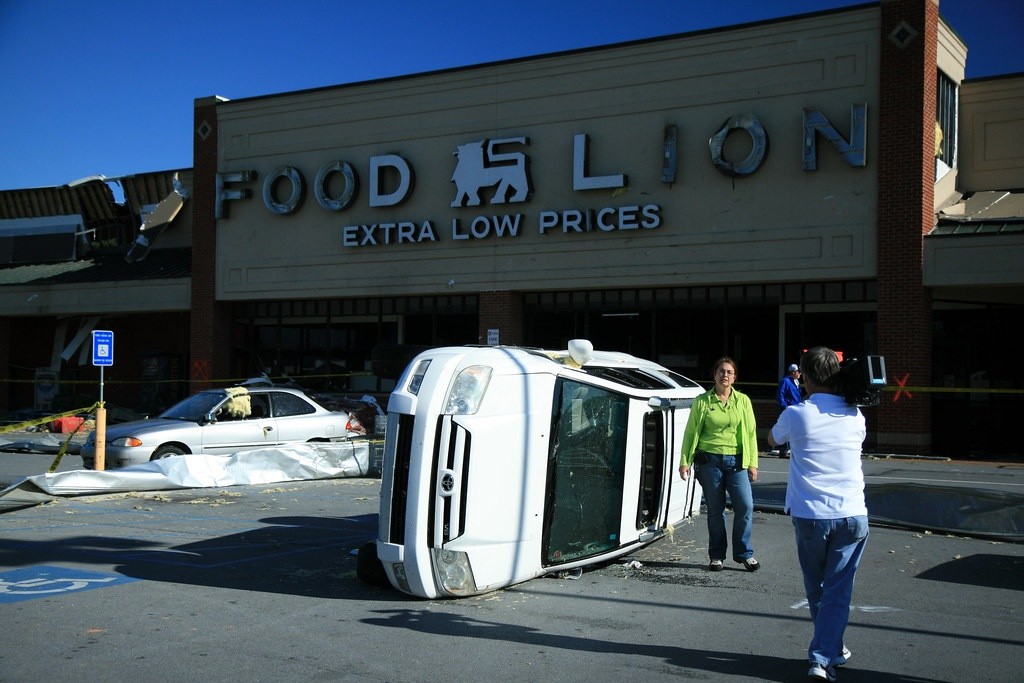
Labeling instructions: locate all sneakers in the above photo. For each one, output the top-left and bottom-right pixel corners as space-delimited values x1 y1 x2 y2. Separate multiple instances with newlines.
708 559 723 571
743 557 760 571
808 662 836 681
830 644 851 667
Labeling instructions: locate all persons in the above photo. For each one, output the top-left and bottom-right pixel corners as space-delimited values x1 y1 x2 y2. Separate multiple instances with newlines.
768 348 868 681
679 357 758 572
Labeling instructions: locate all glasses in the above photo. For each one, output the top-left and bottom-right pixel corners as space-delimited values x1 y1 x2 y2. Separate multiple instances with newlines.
718 368 734 376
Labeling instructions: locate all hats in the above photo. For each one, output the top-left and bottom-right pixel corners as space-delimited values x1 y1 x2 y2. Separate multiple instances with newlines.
789 364 799 371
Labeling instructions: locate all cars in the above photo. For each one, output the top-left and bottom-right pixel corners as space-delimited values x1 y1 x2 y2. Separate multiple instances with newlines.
79 387 351 470
373 338 709 599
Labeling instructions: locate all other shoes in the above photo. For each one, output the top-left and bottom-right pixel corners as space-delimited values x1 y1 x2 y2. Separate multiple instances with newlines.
779 453 790 459
